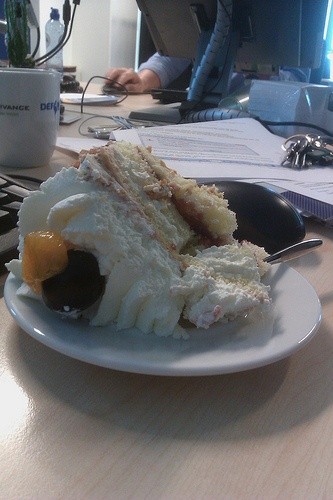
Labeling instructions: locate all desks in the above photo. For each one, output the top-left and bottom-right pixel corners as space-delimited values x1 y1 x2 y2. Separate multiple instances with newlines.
0 83 333 500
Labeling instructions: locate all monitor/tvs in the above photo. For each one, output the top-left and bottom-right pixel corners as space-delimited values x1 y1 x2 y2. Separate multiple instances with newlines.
136 0 328 69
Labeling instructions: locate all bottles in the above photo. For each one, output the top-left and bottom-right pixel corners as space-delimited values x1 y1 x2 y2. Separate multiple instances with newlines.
44 7 64 76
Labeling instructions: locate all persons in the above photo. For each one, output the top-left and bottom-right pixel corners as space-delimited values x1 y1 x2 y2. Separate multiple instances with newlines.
105 51 312 93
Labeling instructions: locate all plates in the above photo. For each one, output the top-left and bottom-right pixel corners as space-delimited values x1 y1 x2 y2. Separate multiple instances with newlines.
4 249 322 375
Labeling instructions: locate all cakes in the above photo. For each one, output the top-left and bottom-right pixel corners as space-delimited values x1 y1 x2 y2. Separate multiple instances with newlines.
4 140 272 339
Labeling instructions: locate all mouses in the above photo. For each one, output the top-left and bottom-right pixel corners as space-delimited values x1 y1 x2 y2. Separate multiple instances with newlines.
103 81 134 95
195 180 306 255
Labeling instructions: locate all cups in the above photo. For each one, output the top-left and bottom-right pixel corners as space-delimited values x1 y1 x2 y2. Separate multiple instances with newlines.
0 69 60 166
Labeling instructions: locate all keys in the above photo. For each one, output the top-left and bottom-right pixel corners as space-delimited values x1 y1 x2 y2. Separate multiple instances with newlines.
278 129 332 171
